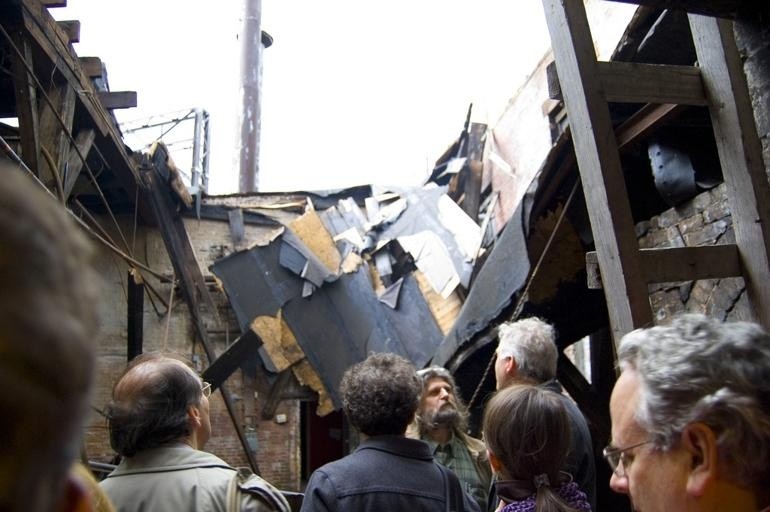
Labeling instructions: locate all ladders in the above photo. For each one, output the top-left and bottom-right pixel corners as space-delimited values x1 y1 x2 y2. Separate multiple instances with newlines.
542 0 770 360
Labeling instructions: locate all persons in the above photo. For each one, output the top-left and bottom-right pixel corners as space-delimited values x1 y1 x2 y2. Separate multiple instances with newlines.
482 385 592 512
0 158 115 512
98 351 291 512
604 313 770 512
300 351 464 512
493 317 595 504
405 365 492 512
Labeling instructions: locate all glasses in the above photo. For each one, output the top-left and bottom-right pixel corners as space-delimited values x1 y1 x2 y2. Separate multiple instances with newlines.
198 381 212 395
604 437 663 474
